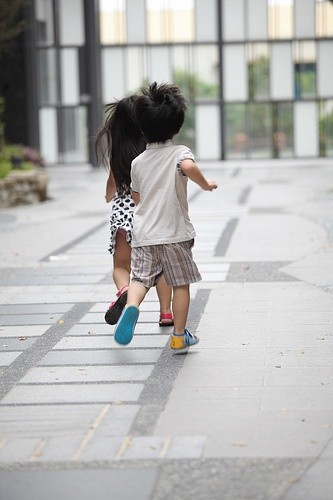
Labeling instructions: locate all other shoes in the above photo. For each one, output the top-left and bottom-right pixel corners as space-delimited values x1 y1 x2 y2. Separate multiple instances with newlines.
159 312 174 326
113 305 140 345
170 329 200 354
105 285 131 326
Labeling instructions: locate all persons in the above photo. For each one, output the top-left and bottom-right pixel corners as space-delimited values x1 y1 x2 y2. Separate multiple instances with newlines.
93 80 218 355
